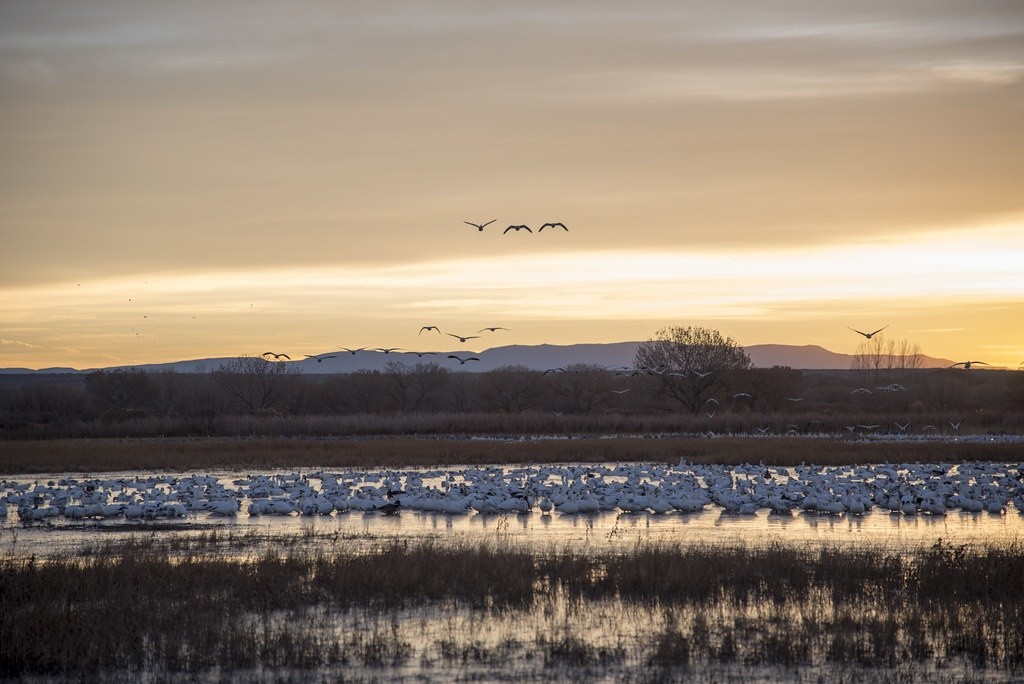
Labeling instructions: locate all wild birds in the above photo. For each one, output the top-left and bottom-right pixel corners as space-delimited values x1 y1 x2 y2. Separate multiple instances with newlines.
445 332 480 343
448 355 480 365
418 326 441 335
846 325 887 338
477 327 509 333
463 219 497 231
263 352 291 361
374 347 402 355
304 353 336 362
1 460 1024 528
538 222 569 232
544 368 566 376
503 224 533 235
461 360 1022 447
404 351 437 358
337 345 369 356
946 360 988 369
